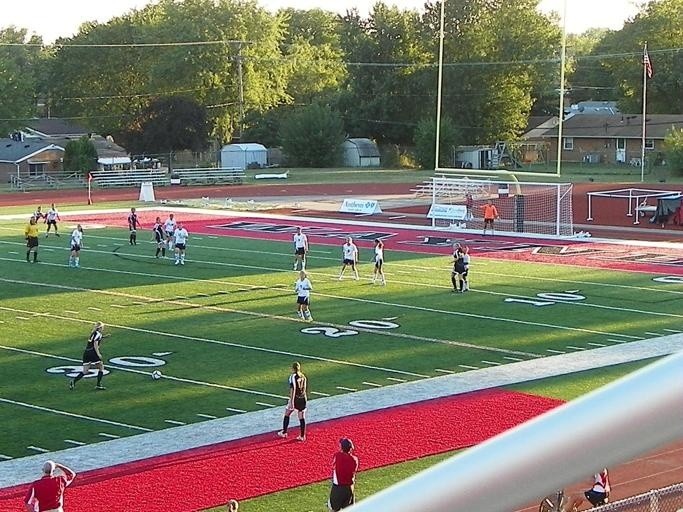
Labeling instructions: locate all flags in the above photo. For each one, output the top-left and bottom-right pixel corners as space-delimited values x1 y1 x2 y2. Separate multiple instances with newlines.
643 45 652 78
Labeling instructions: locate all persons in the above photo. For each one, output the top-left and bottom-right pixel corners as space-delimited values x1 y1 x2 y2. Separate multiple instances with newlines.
227 499 239 512
277 361 307 441
69 321 112 391
295 271 313 323
327 438 359 512
338 237 360 281
24 203 188 269
538 467 611 512
371 239 386 286
448 243 470 292
465 192 474 220
23 460 77 512
293 227 308 271
476 199 501 236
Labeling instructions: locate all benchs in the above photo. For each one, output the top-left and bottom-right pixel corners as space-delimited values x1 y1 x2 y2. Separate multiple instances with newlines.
7 169 90 192
173 167 248 187
410 172 499 199
90 169 171 189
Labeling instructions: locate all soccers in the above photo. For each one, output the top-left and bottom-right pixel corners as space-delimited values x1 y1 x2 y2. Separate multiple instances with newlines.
152 371 161 380
